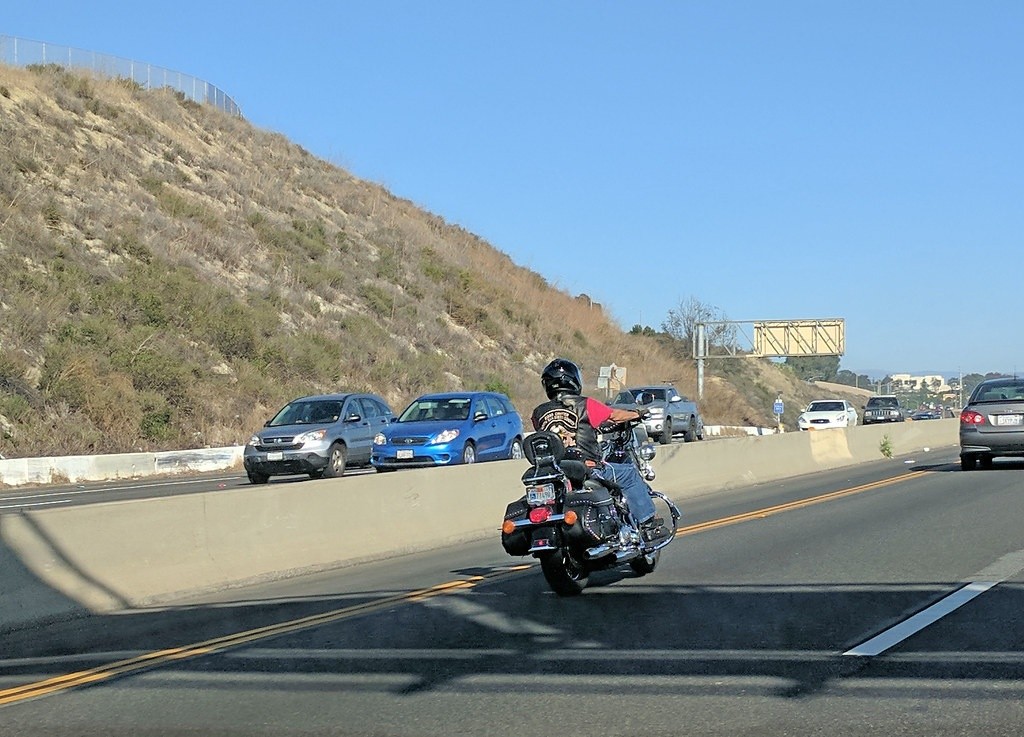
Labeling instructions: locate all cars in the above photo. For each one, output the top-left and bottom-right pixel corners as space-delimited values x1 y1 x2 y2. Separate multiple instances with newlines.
654 414 704 441
902 408 942 421
797 400 859 432
958 377 1023 469
369 391 524 473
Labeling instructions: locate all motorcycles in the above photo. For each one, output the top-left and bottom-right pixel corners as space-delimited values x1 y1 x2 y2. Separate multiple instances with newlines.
500 378 682 598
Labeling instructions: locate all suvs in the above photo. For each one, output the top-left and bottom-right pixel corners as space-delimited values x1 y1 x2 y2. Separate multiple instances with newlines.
860 395 904 425
242 391 400 485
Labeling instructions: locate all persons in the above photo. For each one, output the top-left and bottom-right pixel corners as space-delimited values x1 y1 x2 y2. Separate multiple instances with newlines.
530 359 671 549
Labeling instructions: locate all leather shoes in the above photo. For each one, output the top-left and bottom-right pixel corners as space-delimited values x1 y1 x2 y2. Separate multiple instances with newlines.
642 515 671 542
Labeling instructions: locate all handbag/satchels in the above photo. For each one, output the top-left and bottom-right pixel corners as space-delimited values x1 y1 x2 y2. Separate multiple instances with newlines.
501 496 535 556
562 486 621 549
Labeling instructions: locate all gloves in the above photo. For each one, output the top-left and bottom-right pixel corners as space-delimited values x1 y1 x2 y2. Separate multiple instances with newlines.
635 409 650 420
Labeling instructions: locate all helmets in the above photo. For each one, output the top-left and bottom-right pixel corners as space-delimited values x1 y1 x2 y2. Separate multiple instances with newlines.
541 359 583 400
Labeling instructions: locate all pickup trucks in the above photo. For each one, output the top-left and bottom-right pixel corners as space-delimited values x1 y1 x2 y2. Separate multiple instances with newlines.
603 386 700 446
